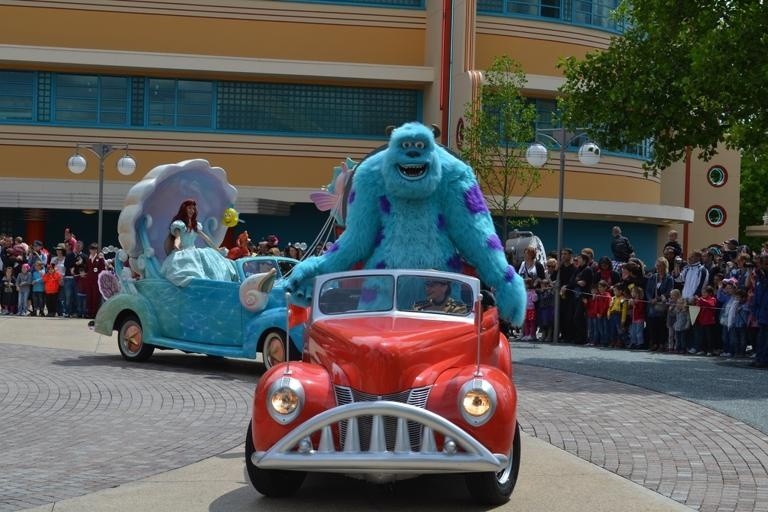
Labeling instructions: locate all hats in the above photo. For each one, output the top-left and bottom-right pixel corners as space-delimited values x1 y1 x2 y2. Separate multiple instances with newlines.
53 243 66 250
724 239 738 246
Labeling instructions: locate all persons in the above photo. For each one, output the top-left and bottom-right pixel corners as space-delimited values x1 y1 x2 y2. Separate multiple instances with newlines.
508 226 768 374
227 224 327 277
413 268 468 314
159 199 237 289
1 227 110 319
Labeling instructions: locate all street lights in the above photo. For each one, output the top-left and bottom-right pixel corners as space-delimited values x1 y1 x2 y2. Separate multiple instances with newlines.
525 128 601 344
67 143 136 256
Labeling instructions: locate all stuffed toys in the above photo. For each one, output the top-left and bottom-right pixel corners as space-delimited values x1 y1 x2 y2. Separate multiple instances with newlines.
285 120 529 329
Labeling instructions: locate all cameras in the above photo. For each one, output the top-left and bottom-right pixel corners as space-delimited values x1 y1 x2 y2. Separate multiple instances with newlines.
676 258 683 265
743 261 755 268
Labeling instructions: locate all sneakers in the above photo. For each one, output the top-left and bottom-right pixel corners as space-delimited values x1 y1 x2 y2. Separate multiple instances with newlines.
516 333 559 342
2 309 96 319
583 339 768 369
